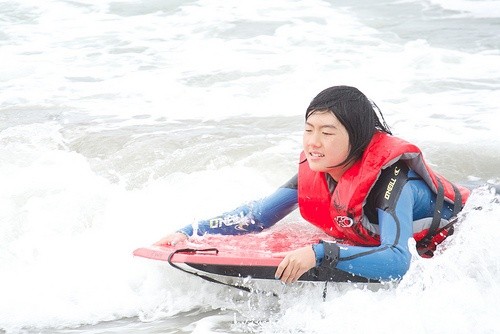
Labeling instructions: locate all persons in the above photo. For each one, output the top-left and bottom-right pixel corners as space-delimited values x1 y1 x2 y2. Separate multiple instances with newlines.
151 85 473 284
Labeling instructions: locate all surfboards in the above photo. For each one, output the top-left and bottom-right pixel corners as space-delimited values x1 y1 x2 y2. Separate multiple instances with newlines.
135 222 382 283
